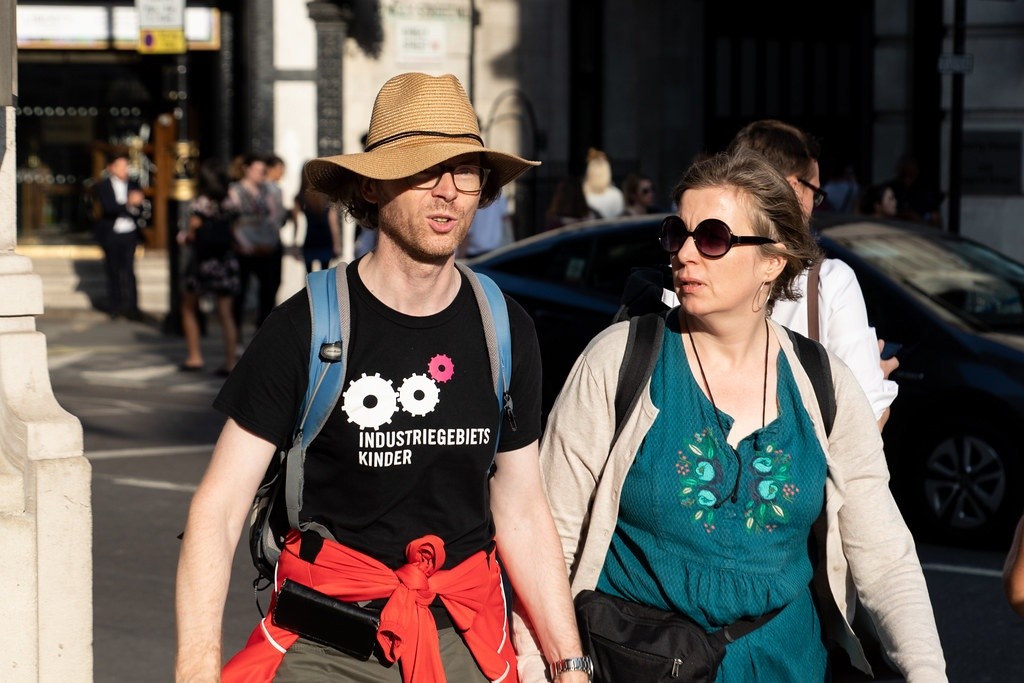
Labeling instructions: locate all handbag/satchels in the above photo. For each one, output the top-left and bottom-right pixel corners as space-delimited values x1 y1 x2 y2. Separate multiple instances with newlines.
570 589 727 683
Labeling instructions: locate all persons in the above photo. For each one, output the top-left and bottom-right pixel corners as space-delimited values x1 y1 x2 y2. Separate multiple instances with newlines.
92 152 343 374
173 74 593 683
540 153 950 683
735 121 898 432
1002 516 1024 619
465 147 678 261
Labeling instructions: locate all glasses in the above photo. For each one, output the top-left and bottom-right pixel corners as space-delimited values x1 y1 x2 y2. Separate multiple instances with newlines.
797 179 827 206
407 161 491 193
656 215 777 259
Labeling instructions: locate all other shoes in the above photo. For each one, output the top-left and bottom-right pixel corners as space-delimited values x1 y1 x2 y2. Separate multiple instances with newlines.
214 367 234 379
179 361 200 372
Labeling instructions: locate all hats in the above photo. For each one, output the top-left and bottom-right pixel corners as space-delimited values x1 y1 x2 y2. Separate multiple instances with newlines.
305 73 543 229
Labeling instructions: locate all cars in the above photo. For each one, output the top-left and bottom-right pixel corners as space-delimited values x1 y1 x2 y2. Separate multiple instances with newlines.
454 210 1024 550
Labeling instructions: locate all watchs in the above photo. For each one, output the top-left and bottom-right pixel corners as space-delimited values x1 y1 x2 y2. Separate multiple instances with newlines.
549 656 594 679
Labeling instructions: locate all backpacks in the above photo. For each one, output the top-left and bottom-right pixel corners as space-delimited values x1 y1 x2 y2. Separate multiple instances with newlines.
250 259 513 580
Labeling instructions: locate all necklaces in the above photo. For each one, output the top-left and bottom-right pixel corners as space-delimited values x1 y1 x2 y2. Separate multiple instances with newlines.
684 315 769 438
